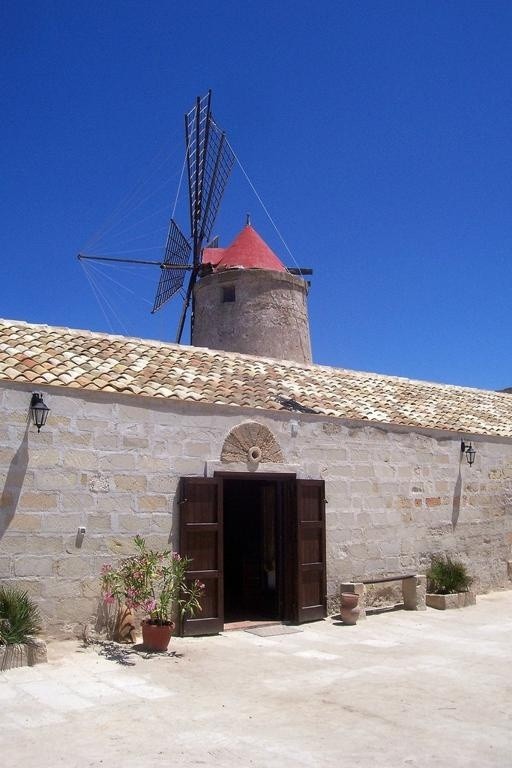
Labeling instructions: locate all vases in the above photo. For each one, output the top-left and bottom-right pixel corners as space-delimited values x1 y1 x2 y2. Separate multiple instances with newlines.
142 619 175 652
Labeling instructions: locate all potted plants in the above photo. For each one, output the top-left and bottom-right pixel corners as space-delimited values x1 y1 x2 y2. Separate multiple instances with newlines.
426 556 477 611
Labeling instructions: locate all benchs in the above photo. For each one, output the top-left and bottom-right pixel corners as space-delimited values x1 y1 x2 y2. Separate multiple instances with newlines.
340 573 426 620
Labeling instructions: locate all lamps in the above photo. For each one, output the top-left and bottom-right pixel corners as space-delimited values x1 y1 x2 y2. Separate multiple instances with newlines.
461 441 476 468
30 393 51 434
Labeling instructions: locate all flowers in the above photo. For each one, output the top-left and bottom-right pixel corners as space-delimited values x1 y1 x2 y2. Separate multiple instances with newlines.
100 534 208 628
